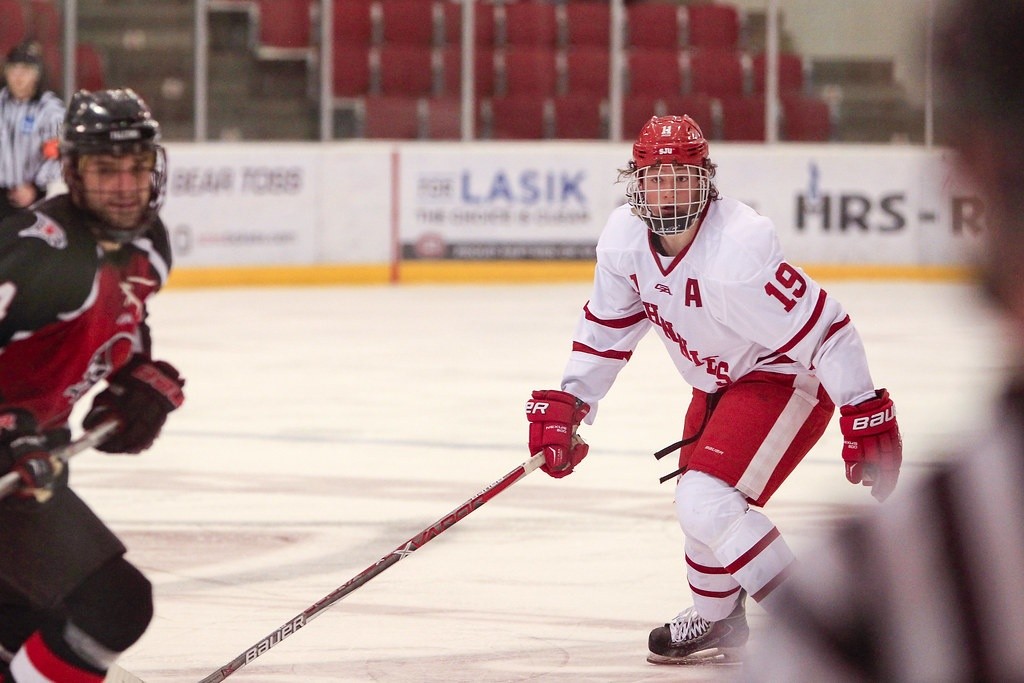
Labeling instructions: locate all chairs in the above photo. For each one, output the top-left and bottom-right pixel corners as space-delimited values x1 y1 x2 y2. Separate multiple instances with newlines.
0 0 105 99
208 1 832 144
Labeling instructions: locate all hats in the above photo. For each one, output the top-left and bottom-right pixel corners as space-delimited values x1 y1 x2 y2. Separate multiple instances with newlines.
7 39 43 67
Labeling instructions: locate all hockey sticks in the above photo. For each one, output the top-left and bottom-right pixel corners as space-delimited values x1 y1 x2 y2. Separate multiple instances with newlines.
195 434 585 683
0 422 116 496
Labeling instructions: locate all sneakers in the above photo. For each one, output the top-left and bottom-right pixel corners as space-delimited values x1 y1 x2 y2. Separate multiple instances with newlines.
647 590 747 670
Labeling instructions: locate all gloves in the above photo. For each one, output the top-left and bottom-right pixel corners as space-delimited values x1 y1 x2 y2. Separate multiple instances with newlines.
83 357 186 454
526 388 590 478
1 411 72 507
840 389 903 503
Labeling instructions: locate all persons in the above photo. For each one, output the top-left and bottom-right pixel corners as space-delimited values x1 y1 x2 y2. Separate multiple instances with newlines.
0 37 70 214
525 113 902 667
748 1 1024 682
0 83 184 683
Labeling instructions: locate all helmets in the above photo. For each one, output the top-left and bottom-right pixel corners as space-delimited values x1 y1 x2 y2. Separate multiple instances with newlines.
62 87 169 243
624 116 714 235
